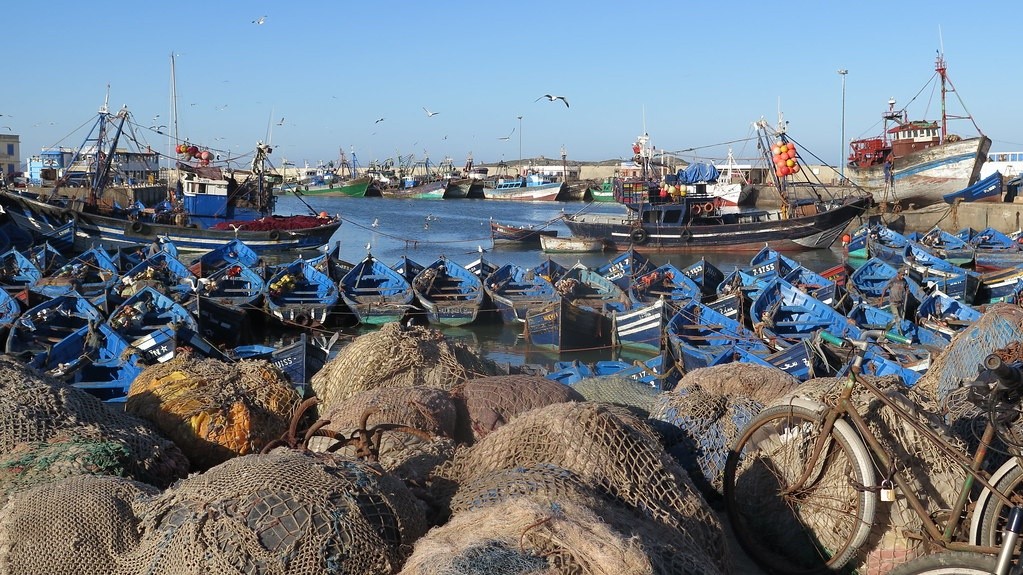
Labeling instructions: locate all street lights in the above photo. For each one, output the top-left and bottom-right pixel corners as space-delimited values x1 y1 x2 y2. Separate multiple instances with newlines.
836 69 849 186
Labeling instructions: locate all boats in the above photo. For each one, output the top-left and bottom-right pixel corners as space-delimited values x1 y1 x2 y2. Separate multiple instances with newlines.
539 234 609 253
482 169 565 201
846 24 992 207
488 221 559 246
379 178 450 200
299 176 372 197
943 170 1003 205
0 95 1022 406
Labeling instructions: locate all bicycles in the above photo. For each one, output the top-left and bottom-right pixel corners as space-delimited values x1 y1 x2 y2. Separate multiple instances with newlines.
723 329 1022 575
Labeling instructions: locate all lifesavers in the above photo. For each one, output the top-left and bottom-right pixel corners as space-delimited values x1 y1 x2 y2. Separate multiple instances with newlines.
631 229 647 244
705 203 713 212
59 209 78 225
294 314 308 325
680 230 691 241
305 186 308 190
48 159 52 164
132 221 142 232
269 230 279 240
692 205 701 214
329 185 333 189
144 203 150 208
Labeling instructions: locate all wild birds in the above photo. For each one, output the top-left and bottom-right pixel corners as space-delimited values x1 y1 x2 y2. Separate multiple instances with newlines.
149 125 166 130
319 332 340 361
158 232 170 244
423 107 440 118
364 243 372 251
0 114 13 132
574 215 585 222
535 94 569 107
277 117 284 125
498 128 515 142
190 81 231 141
371 214 535 257
185 278 211 294
375 118 387 123
443 135 448 141
324 244 330 251
229 224 246 238
251 16 268 25
